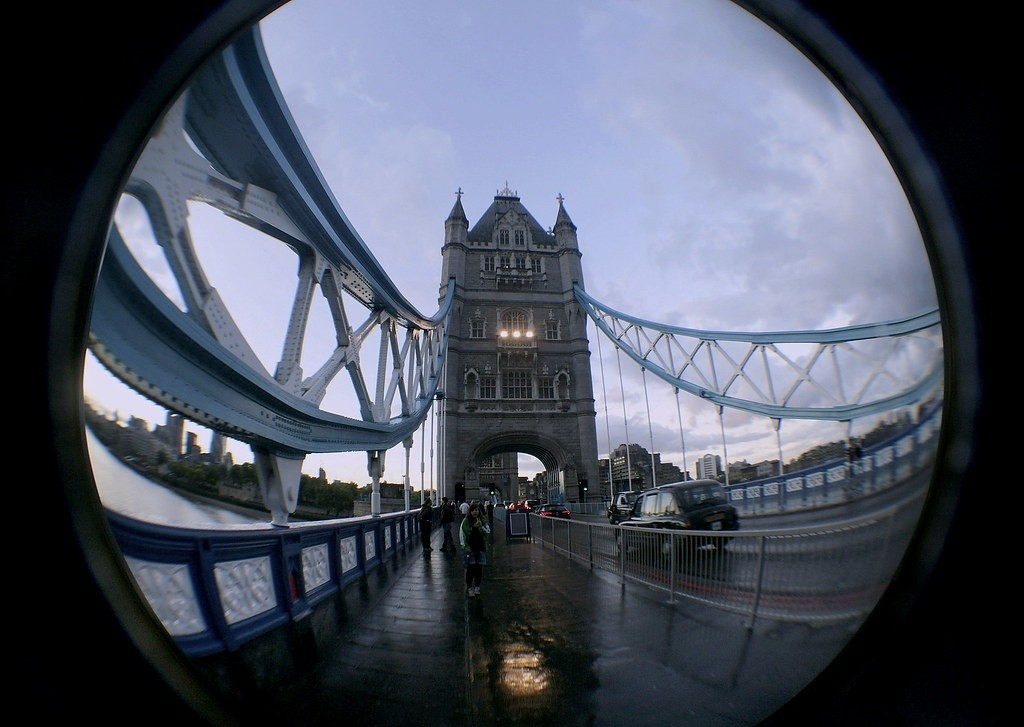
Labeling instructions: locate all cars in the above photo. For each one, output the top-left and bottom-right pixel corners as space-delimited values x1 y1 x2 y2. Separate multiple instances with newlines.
509 503 524 511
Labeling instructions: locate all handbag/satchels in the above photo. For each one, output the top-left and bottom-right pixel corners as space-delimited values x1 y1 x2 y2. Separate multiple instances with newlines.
468 527 487 552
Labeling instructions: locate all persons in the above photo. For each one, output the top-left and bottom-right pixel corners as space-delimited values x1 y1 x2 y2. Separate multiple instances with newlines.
846 437 862 478
438 497 457 553
521 501 525 508
492 494 500 502
448 500 486 518
417 497 433 551
487 501 494 523
459 502 491 597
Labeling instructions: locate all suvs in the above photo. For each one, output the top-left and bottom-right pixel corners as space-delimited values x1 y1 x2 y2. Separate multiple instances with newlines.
616 479 739 559
494 503 504 509
535 504 572 527
524 500 539 511
607 490 644 524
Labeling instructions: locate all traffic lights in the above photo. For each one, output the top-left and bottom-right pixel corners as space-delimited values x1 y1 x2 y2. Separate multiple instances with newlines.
489 482 495 495
582 479 588 491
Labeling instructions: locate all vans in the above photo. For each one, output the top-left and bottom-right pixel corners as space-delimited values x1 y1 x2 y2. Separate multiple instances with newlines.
504 501 514 509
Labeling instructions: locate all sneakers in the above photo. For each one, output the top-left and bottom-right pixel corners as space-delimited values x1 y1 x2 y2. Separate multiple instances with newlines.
474 586 480 594
466 587 475 596
